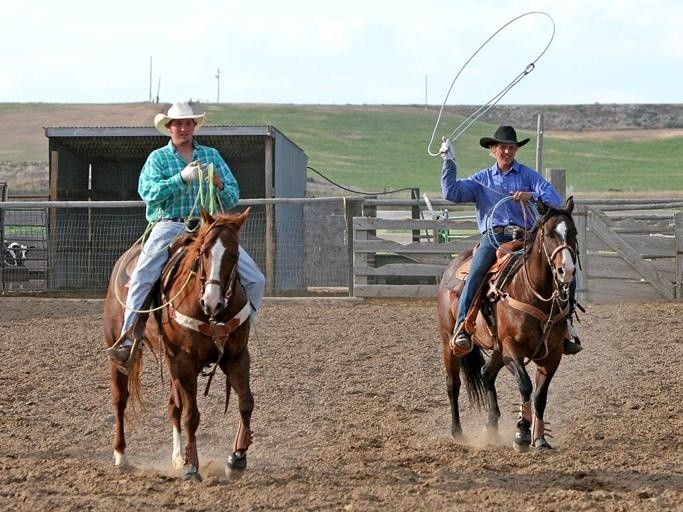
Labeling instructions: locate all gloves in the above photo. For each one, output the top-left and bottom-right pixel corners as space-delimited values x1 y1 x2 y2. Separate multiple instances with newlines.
180 161 211 186
439 136 457 161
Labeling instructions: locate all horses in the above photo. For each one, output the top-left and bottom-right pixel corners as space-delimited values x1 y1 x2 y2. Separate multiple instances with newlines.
435 194 579 454
102 203 256 486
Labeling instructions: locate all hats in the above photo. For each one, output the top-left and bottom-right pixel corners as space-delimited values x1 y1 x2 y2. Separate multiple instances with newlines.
479 125 532 149
153 102 206 136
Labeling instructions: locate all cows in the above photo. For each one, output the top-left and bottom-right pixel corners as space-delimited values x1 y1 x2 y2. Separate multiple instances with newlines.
0 239 37 268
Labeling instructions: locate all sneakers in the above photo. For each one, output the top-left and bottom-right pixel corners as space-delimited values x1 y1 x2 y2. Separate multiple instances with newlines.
564 332 584 356
446 332 471 358
106 345 134 376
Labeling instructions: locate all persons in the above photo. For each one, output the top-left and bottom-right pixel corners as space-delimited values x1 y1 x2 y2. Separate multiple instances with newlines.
439 126 582 356
107 101 266 376
